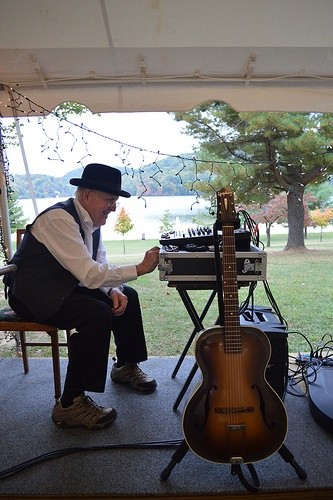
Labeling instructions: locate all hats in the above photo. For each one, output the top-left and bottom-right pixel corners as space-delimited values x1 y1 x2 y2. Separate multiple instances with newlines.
69 163 131 198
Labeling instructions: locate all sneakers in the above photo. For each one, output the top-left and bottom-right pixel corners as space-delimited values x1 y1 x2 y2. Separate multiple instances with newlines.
110 357 157 392
51 393 118 430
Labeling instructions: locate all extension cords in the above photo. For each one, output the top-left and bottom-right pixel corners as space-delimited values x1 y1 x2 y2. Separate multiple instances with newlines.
295 355 333 366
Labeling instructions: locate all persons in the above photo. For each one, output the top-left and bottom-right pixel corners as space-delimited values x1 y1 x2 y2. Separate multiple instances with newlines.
3 164 160 431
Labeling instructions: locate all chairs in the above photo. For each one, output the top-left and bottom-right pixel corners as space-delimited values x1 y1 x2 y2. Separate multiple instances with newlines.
0 228 72 400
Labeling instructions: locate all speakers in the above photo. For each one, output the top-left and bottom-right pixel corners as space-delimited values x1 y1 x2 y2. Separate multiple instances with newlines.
240 305 289 403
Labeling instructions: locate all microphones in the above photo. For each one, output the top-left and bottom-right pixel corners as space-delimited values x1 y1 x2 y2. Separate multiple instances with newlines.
0 264 17 276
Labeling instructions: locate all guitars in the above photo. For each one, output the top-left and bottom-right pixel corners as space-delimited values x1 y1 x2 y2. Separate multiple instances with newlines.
181 187 288 464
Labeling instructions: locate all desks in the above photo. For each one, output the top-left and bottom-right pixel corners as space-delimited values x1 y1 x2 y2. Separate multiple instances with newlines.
166 281 248 410
